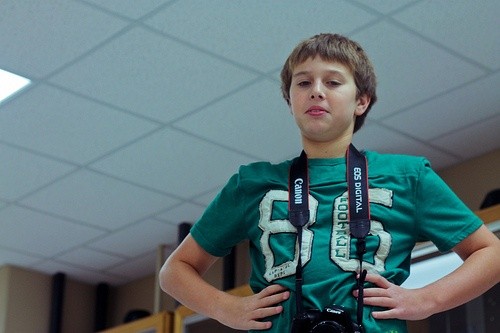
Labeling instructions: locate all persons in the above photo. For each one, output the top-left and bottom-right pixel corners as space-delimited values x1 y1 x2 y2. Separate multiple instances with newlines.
159 33 500 333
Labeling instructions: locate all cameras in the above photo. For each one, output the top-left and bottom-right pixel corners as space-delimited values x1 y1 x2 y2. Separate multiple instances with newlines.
290 304 364 333
